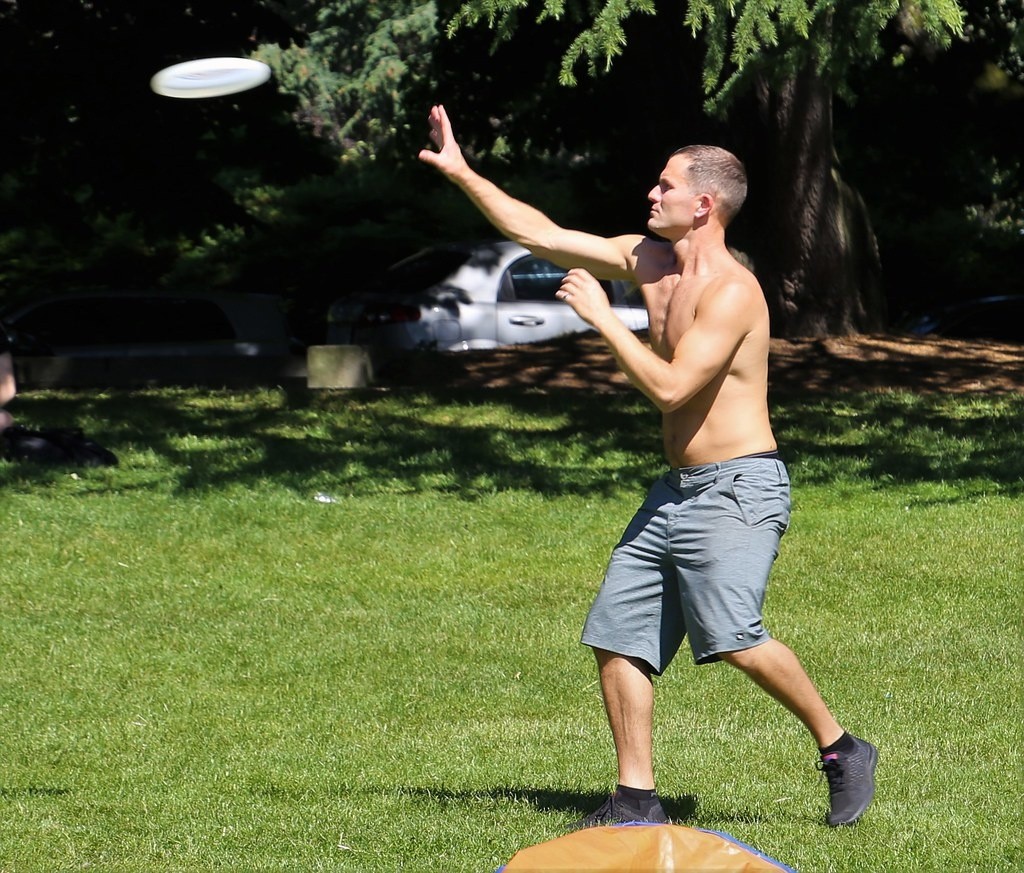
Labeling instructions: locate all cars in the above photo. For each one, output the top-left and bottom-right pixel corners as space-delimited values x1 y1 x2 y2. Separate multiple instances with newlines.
377 240 651 354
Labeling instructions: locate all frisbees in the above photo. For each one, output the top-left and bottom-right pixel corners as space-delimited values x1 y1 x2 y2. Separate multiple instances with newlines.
151 55 271 99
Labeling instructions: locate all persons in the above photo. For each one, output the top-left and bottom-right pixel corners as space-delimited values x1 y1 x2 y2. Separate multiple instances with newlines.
420 105 879 835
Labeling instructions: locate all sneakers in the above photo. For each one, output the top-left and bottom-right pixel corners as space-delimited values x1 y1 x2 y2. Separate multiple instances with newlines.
563 792 670 829
816 734 878 830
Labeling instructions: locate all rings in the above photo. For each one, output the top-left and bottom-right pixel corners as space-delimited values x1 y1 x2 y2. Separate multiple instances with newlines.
561 293 570 302
